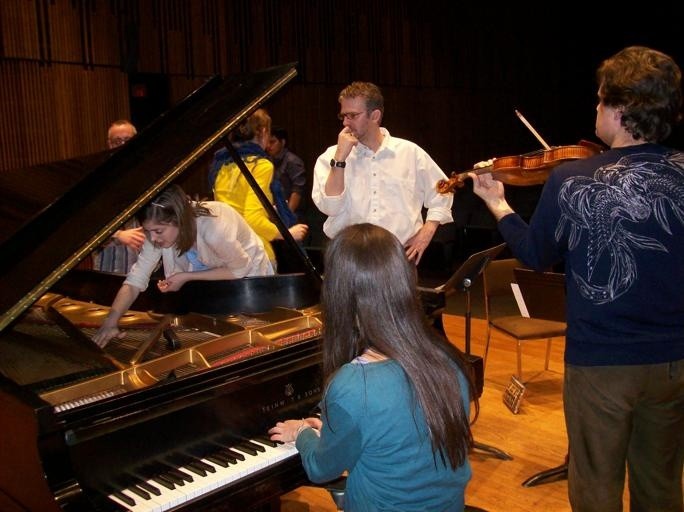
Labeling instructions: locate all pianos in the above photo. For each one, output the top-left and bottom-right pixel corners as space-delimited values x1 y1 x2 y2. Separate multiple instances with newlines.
1 68 484 511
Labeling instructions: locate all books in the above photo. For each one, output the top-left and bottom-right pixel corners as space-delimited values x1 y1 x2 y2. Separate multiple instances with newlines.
503 375 526 414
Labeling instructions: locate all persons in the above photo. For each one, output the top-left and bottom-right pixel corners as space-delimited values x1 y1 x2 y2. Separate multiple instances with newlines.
268 223 480 512
467 46 684 512
311 81 456 265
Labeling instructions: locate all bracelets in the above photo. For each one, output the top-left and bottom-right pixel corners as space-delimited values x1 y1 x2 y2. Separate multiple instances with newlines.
295 425 312 441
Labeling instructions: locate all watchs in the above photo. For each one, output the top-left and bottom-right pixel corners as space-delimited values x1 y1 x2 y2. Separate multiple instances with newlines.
330 158 346 168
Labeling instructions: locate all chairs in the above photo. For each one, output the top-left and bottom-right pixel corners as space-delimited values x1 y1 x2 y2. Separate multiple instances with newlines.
482 257 568 382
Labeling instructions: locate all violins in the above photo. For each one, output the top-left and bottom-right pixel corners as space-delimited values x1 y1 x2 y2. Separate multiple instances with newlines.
436 141 602 193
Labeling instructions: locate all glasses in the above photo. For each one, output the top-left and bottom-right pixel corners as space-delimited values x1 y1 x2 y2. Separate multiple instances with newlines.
338 112 364 120
114 136 130 144
